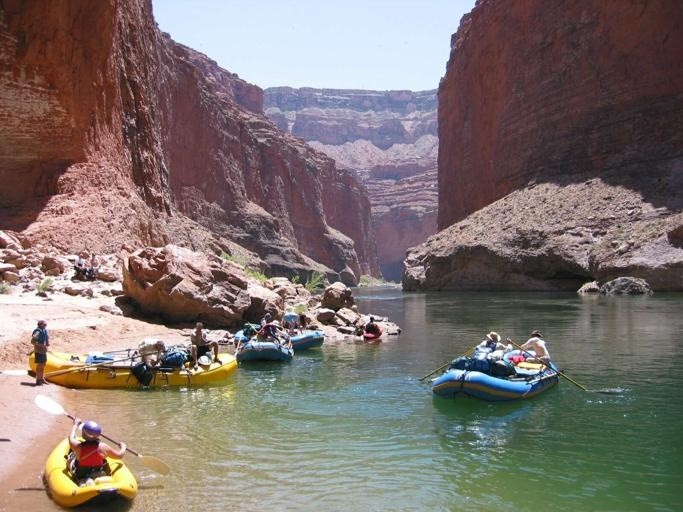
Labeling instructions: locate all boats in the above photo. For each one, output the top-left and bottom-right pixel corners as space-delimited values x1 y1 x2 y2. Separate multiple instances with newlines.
29 350 238 389
233 326 324 363
44 437 138 508
432 348 559 402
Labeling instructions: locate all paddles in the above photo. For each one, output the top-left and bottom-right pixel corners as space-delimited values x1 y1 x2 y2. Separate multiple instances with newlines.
43 350 167 379
35 396 169 475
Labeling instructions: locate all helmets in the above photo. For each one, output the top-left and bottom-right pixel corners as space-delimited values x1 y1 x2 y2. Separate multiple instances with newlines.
82 421 101 440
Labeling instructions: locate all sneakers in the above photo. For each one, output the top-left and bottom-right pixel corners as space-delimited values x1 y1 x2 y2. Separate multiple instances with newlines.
36 378 49 386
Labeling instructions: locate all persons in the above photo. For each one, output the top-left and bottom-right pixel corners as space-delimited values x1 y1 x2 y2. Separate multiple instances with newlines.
365 315 379 334
136 336 166 367
66 417 128 479
190 321 223 369
242 310 308 349
73 253 87 281
518 329 551 364
475 330 513 361
88 253 99 281
30 317 49 385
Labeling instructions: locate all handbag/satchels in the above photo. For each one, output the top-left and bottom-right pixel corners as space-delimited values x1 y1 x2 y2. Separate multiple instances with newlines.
130 362 153 386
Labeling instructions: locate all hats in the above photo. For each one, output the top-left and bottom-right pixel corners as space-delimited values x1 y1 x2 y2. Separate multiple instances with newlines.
531 330 544 338
486 330 502 342
